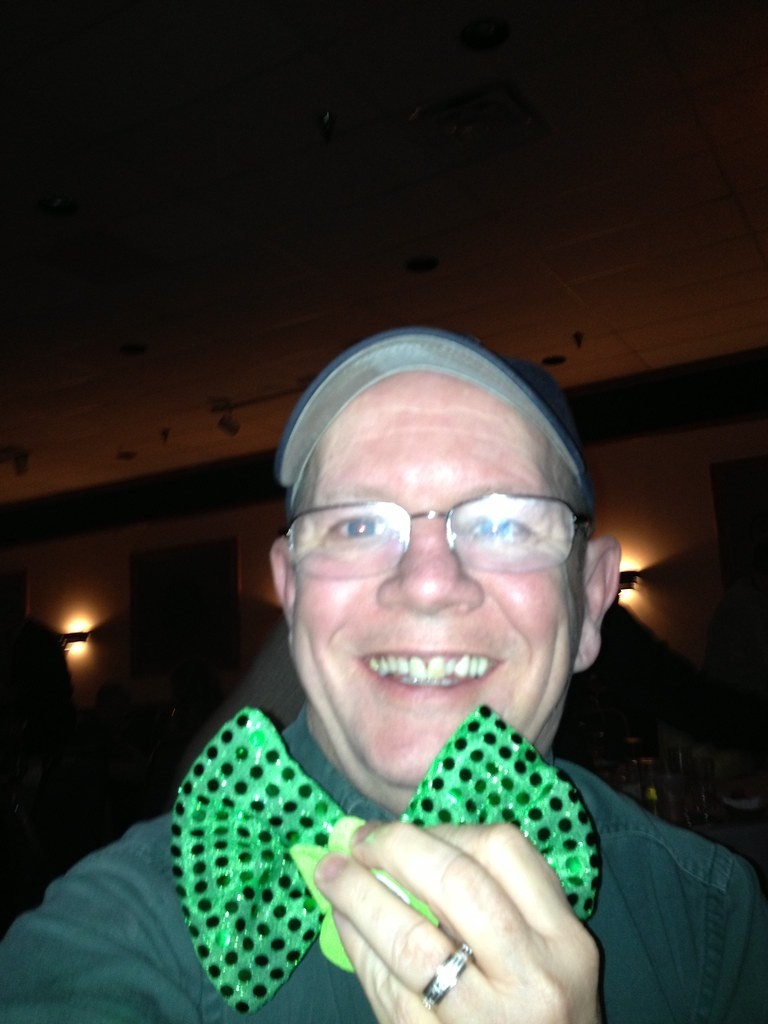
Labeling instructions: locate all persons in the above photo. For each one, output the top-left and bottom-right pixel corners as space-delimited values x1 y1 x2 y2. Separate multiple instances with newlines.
0 327 768 1024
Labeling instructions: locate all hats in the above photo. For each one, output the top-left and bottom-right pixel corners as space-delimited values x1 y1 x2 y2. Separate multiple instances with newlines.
271 323 592 507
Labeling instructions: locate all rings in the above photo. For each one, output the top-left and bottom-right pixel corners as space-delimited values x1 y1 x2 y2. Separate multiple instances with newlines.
420 943 474 1009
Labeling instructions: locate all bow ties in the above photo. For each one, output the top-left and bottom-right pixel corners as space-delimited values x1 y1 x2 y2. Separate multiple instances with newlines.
170 702 604 1017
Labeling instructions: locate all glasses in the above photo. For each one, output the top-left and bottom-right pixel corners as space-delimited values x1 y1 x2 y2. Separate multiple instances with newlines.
282 492 596 582
